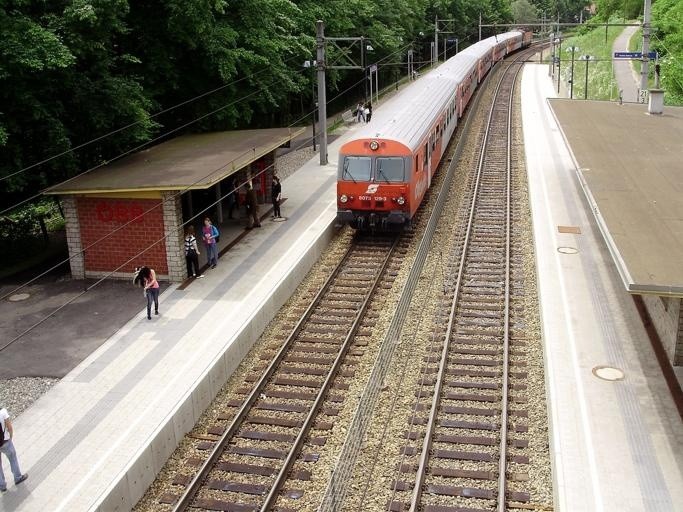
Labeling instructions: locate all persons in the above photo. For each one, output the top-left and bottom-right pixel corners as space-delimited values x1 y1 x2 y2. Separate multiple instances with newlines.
227 177 237 220
197 218 219 269
133 266 160 320
271 176 282 218
184 225 205 279
365 102 372 124
357 101 365 123
0 407 29 491
243 183 261 230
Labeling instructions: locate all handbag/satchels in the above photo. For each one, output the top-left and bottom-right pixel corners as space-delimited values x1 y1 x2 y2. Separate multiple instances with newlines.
187 247 197 259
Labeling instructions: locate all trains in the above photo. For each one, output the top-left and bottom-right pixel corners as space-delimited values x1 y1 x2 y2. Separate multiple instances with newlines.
335 30 537 234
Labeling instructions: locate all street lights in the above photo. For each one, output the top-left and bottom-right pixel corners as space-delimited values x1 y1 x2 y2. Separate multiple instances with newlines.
547 32 594 100
303 59 318 152
362 41 373 102
410 31 423 77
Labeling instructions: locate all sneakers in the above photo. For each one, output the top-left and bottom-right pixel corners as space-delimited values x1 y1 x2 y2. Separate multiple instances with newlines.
244 222 261 231
189 273 205 279
210 263 217 269
13 472 29 485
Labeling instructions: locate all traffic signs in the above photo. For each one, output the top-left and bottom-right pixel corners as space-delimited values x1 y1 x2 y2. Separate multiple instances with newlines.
615 53 657 59
